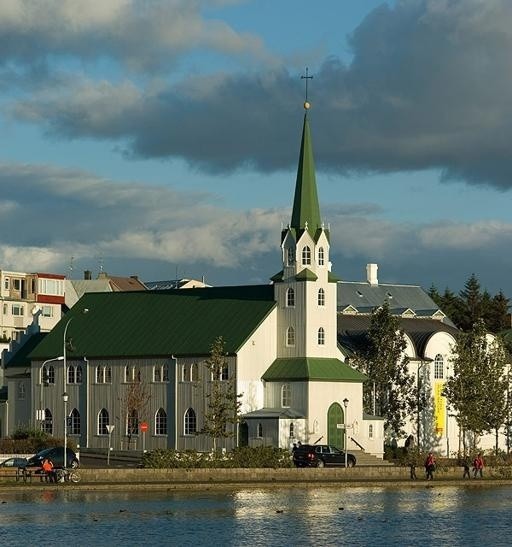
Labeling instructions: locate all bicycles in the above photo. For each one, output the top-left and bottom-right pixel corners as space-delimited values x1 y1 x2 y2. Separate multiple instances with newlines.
54 465 82 483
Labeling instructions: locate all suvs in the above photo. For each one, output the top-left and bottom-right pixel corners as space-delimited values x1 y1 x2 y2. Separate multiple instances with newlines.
292 444 356 468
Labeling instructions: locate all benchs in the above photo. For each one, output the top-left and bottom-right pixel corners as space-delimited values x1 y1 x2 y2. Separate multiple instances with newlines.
0 466 57 484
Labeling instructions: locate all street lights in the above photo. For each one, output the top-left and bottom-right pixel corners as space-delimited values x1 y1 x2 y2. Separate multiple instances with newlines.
60 307 91 469
37 355 64 435
62 391 71 470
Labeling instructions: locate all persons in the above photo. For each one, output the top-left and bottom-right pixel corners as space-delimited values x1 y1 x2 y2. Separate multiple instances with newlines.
425 452 436 480
42 457 58 483
472 453 483 479
463 455 470 478
409 460 418 479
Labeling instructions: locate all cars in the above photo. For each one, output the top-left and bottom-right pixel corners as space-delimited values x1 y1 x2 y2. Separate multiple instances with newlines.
25 447 82 470
0 457 28 469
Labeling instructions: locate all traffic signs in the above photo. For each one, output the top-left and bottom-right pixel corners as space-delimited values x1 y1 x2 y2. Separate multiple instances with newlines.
343 398 349 469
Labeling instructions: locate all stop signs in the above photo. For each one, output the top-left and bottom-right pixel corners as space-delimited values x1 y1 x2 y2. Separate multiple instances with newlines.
140 422 149 432
